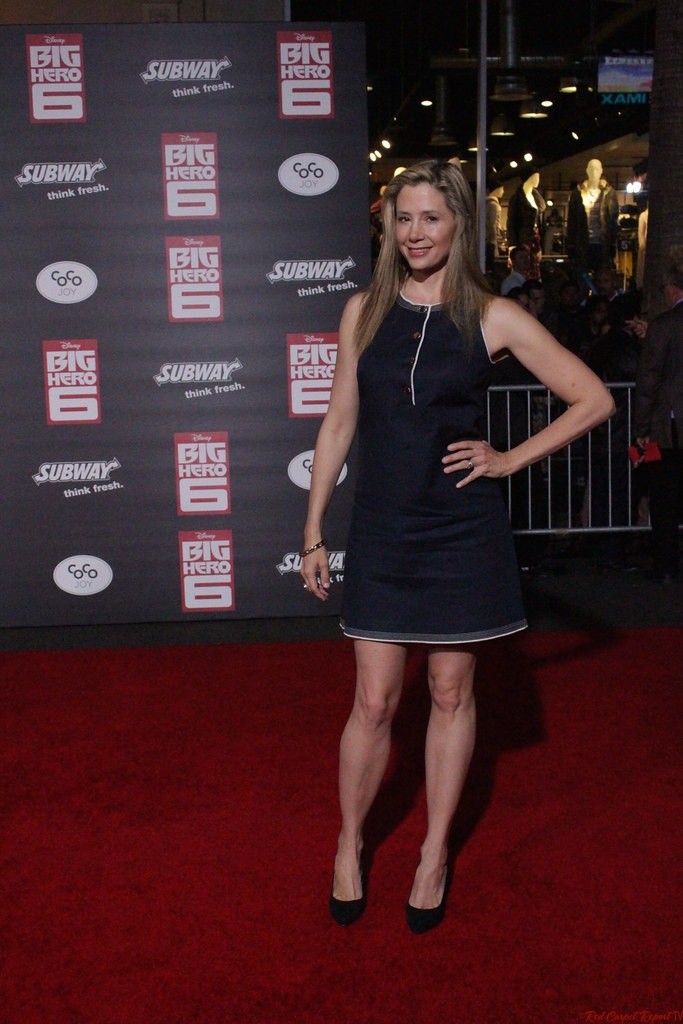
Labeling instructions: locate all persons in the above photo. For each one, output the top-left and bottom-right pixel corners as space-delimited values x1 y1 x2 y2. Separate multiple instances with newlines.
299 160 615 934
624 262 683 578
370 186 388 241
485 177 505 256
485 242 642 578
505 166 546 280
567 157 619 297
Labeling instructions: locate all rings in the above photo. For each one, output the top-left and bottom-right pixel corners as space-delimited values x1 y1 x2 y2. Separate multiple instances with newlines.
304 584 310 587
467 460 474 469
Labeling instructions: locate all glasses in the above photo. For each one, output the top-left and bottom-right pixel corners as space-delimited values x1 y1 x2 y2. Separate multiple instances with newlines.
658 283 672 295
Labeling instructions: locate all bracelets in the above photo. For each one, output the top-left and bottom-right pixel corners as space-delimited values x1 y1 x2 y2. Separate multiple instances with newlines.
299 539 327 557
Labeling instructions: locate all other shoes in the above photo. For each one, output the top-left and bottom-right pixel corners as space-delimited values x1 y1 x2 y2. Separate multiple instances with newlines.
329 873 365 925
406 866 450 934
